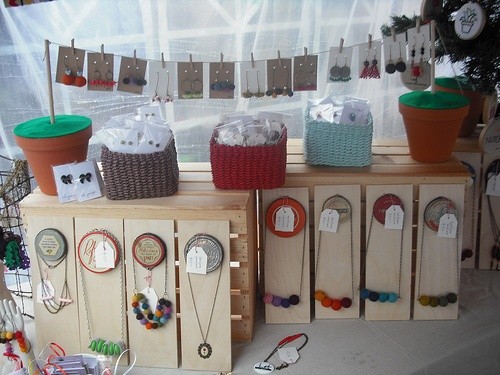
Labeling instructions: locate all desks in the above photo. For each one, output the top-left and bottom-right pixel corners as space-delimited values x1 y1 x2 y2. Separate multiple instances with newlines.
259 139 472 276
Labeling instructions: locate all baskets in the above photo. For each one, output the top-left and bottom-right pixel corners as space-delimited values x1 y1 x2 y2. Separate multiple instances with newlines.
210 122 287 190
303 106 373 167
101 137 179 201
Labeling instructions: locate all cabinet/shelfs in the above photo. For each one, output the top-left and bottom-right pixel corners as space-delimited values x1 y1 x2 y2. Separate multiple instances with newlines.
19 162 256 342
